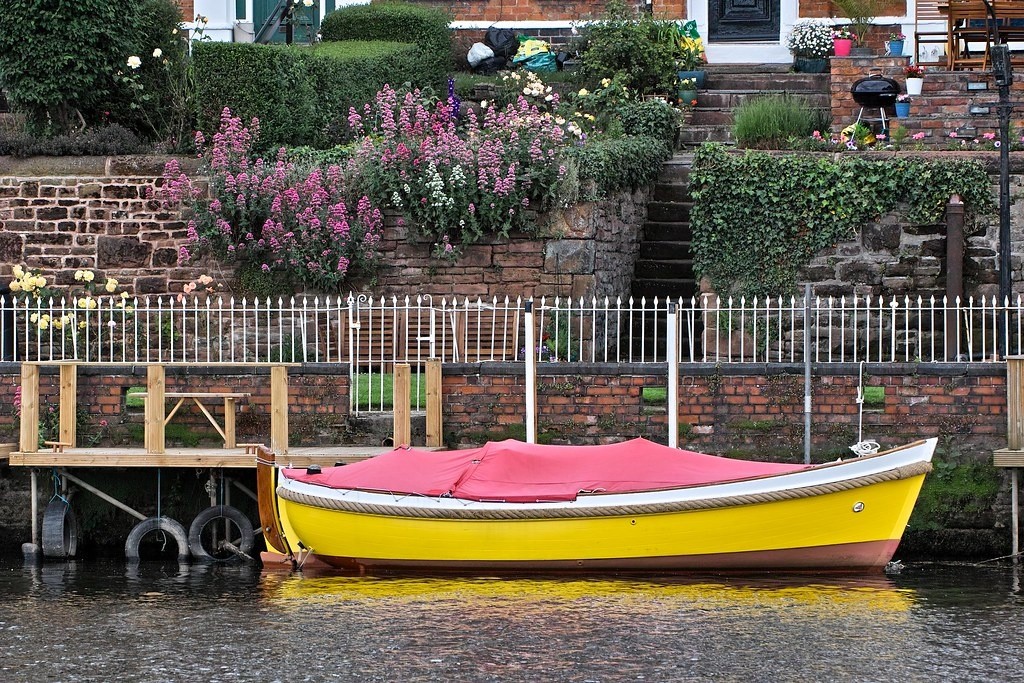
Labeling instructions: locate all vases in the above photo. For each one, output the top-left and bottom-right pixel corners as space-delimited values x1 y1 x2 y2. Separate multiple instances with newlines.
906 77 924 95
797 58 826 73
896 102 910 118
833 39 852 56
685 111 694 124
678 88 698 105
890 41 904 55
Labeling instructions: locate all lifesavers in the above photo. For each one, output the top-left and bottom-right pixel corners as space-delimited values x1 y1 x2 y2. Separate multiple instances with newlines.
124 515 190 564
41 497 81 558
186 504 255 561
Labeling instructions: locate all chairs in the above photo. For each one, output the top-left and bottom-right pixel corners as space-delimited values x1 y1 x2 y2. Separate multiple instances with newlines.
915 0 1024 70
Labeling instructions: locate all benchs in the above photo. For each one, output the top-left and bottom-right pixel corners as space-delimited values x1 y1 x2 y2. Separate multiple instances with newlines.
128 391 251 450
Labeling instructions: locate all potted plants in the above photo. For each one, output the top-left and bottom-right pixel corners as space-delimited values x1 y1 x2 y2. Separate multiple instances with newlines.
831 0 892 56
678 47 705 87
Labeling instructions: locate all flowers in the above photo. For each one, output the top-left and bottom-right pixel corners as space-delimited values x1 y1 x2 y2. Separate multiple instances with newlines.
885 32 907 41
677 98 698 113
903 64 928 78
784 20 835 60
895 93 912 103
830 26 858 42
673 76 697 90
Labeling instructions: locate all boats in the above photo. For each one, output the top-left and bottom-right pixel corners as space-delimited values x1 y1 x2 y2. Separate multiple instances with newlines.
276 436 938 578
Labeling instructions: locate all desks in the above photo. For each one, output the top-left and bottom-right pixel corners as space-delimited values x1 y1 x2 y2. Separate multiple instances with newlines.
933 4 962 71
